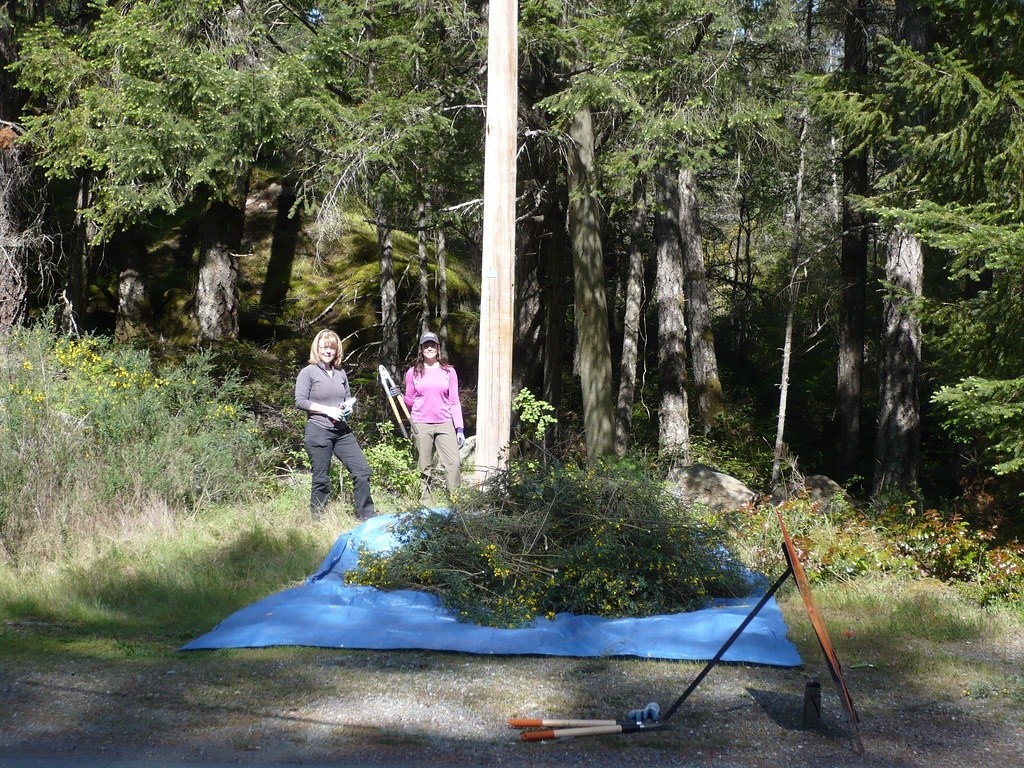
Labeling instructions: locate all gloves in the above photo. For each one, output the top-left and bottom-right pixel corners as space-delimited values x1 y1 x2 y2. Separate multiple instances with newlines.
628 702 660 721
389 385 401 397
339 402 353 417
456 433 465 449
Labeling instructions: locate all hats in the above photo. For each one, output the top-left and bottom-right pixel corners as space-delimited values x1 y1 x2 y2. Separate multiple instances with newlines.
419 331 439 345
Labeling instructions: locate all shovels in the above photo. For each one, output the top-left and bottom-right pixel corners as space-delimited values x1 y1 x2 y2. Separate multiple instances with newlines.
509 708 677 743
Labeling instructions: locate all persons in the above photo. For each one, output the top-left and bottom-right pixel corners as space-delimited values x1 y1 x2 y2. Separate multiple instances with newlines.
390 332 465 506
295 329 379 521
